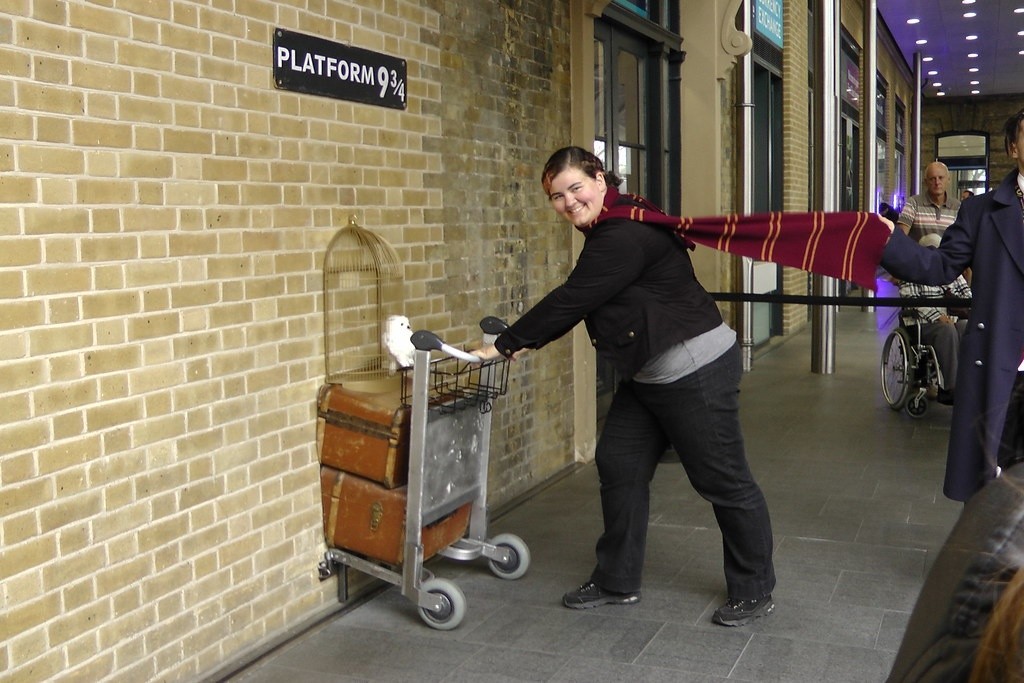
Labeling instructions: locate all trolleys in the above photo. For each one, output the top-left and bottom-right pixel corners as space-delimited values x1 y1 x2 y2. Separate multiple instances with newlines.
327 316 530 630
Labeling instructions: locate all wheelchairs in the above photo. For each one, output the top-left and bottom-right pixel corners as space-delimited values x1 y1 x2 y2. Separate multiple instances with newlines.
881 308 956 418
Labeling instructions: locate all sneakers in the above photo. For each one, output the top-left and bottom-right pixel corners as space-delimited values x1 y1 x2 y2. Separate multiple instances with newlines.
563 580 642 609
711 592 775 627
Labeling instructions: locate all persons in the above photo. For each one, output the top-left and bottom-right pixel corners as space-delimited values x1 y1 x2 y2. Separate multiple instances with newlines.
877 107 1024 503
879 202 899 222
960 190 975 201
883 156 960 244
467 147 777 627
899 232 974 405
